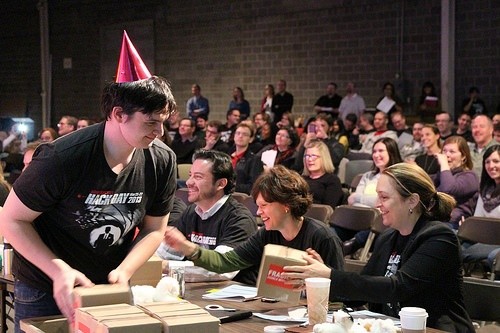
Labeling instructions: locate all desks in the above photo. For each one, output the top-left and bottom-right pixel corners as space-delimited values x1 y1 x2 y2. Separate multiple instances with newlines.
20 258 448 333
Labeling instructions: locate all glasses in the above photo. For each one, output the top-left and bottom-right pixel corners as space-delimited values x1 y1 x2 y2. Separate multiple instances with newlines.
57 123 69 127
305 154 321 159
232 112 240 117
276 132 290 139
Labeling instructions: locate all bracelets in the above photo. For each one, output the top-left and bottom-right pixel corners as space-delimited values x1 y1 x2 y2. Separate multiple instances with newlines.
185 244 200 260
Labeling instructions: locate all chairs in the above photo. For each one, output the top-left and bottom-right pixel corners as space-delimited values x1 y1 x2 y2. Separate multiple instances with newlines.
176 150 500 325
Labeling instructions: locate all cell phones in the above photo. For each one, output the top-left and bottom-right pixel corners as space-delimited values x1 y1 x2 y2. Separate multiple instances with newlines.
261 297 279 303
307 123 316 134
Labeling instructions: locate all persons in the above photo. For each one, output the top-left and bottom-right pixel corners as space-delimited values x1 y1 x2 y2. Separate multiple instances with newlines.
328 138 405 257
450 144 500 280
0 76 177 333
167 148 261 290
299 138 345 210
0 80 500 172
163 164 346 275
429 137 480 231
281 162 476 333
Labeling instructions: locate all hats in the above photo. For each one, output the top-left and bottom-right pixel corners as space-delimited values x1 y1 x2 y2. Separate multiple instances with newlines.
116 29 152 84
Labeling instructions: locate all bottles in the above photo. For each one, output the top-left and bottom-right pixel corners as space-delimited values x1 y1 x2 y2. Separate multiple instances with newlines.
3 238 13 276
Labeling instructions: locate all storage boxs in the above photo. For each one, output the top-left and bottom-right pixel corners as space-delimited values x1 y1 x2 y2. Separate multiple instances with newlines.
258 244 310 304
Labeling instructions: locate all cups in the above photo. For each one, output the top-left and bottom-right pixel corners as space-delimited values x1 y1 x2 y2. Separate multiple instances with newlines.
305 279 332 326
168 268 185 299
398 307 429 333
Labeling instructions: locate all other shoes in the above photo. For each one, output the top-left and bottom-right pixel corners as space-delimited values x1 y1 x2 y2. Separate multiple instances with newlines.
342 237 361 257
